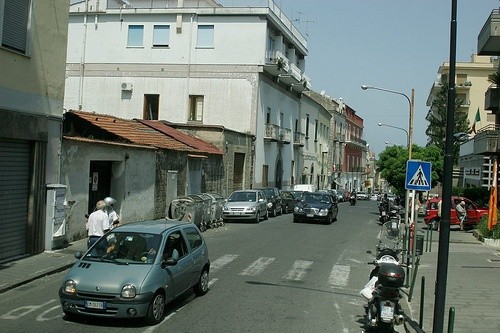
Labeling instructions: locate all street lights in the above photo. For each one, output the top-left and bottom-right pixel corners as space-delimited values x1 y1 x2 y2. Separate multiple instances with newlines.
361 85 415 265
377 123 409 160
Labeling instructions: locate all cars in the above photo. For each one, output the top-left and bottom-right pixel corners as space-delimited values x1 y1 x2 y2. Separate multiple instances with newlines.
318 189 351 203
280 190 298 214
292 192 339 225
221 189 269 223
290 191 304 203
58 218 211 326
356 191 369 200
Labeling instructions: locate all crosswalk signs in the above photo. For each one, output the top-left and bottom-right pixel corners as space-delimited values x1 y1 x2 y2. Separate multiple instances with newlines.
405 160 431 191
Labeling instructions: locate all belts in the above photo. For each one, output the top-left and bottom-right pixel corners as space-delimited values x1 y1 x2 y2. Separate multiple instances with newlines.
90 236 101 238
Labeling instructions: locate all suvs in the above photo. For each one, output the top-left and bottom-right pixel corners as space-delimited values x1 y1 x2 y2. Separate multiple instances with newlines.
251 187 283 218
423 196 489 231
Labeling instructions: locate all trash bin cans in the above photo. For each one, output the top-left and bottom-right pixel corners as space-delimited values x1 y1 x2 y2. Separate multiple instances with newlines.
170 192 224 233
416 230 425 255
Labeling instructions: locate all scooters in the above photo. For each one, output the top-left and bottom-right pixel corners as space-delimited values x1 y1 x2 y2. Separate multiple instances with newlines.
348 193 357 206
358 188 414 333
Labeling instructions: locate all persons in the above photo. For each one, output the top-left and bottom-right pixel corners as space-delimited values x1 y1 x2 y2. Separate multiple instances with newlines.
107 242 119 259
103 197 119 246
382 194 389 210
86 200 110 256
352 188 356 197
456 201 467 231
437 200 442 232
149 235 168 260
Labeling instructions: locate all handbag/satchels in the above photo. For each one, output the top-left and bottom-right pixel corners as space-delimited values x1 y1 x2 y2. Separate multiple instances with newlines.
360 276 378 301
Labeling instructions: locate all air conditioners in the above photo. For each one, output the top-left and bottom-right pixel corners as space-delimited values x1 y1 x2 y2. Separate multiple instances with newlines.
121 82 134 90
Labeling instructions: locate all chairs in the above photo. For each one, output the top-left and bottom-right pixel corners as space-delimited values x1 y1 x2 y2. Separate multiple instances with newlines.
247 194 255 198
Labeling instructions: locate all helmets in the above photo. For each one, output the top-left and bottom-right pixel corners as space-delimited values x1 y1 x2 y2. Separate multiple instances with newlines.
96 200 106 209
104 197 117 207
